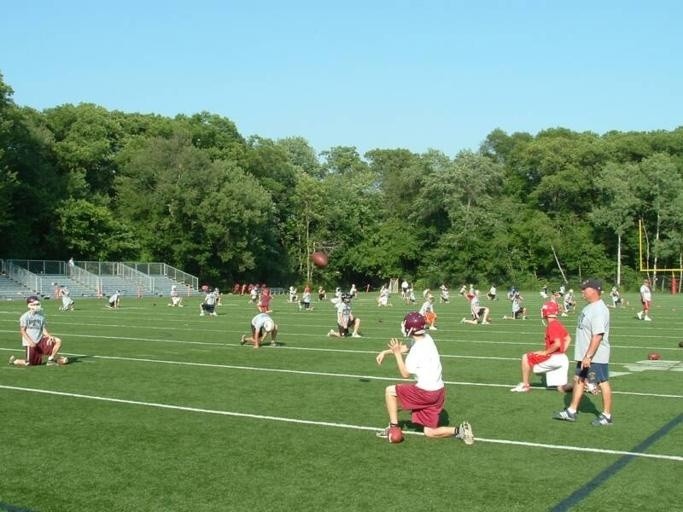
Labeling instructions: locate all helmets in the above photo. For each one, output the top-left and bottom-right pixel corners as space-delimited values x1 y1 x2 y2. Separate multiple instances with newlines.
27 296 42 313
541 302 559 319
341 294 352 304
264 321 274 332
401 313 425 338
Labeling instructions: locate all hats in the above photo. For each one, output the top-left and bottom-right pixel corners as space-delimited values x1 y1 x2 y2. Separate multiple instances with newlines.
579 279 602 291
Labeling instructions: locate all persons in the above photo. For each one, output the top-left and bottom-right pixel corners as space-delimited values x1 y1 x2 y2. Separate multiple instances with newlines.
637 279 651 321
511 302 597 394
459 284 529 323
552 280 611 425
59 285 75 311
105 289 122 309
607 286 622 309
376 282 450 330
543 282 576 313
168 286 184 308
376 311 473 445
290 284 363 338
198 284 280 349
9 296 62 367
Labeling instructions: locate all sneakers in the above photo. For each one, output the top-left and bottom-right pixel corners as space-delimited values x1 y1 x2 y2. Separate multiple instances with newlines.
47 360 59 367
510 383 530 392
8 355 16 366
327 329 334 337
352 333 361 338
553 409 576 421
240 335 246 345
270 343 280 347
586 379 598 395
459 421 474 445
376 424 401 438
592 413 613 425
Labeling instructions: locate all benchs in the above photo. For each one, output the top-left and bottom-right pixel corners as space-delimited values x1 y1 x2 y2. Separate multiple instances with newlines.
0 269 202 301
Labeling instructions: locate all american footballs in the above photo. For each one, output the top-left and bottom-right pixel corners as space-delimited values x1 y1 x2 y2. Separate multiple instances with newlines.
564 385 572 391
313 253 330 267
54 356 69 365
388 427 404 444
648 352 661 360
